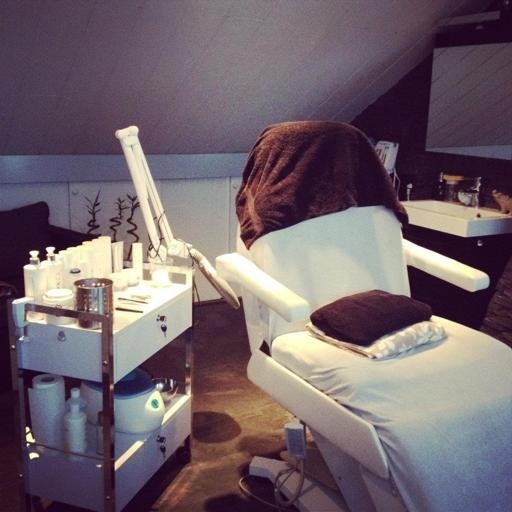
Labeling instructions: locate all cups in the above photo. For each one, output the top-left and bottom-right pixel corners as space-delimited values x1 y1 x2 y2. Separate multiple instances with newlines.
149 256 173 289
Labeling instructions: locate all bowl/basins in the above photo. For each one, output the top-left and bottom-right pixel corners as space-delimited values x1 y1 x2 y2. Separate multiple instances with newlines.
151 377 179 403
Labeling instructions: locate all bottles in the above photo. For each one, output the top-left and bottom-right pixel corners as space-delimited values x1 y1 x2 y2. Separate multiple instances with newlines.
63 404 86 459
96 411 104 455
65 388 86 416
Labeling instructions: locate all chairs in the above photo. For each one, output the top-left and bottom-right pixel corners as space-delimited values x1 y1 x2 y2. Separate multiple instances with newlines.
212 119 512 508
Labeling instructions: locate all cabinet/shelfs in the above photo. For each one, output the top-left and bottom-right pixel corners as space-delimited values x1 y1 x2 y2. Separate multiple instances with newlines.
5 260 196 512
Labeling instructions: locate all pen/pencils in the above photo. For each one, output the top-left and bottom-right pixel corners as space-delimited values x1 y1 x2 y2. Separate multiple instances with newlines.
116 307 143 313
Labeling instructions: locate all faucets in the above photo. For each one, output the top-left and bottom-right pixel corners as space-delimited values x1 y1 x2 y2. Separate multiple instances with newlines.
406 181 414 202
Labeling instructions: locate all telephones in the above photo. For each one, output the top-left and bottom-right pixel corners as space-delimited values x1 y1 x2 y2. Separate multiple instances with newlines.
374 141 399 175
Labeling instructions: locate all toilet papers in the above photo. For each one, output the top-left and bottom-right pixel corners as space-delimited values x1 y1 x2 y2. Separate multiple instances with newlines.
27 374 66 457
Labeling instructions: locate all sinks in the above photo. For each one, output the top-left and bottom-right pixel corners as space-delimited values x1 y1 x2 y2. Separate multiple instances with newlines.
406 201 506 221
